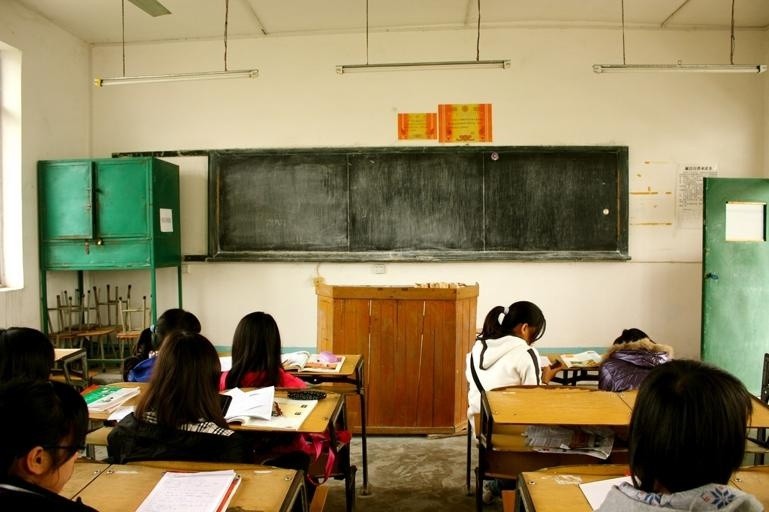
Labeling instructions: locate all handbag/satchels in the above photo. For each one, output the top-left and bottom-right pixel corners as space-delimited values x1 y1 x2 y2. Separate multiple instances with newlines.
271 431 352 485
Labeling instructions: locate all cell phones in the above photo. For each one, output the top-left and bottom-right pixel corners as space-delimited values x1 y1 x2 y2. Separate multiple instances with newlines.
549 358 562 370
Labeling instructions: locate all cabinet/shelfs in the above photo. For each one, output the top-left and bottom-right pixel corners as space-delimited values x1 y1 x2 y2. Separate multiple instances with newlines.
37 157 182 364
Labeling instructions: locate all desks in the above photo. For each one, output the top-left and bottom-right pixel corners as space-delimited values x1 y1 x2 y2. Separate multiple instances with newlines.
55 352 368 512
464 347 768 510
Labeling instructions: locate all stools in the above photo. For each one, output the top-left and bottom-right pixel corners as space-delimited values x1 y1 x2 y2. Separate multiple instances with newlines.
47 285 151 373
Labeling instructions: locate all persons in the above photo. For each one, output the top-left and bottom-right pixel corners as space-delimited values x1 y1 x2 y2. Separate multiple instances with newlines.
0 379 98 512
465 301 564 506
598 327 675 391
594 359 764 511
122 309 201 382
0 328 55 385
218 311 308 391
107 331 317 512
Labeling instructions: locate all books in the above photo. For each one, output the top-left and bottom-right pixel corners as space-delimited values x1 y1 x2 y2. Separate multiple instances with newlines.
221 386 318 431
279 351 345 373
559 351 604 368
79 384 140 413
134 470 241 512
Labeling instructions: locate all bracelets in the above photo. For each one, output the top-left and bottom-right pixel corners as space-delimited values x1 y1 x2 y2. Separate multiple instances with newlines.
542 380 549 386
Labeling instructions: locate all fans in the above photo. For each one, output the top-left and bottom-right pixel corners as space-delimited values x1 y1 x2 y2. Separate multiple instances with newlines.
128 0 172 17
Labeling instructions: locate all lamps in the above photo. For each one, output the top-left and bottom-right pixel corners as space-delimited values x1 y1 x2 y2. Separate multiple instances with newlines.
336 0 511 74
92 0 258 87
592 0 767 73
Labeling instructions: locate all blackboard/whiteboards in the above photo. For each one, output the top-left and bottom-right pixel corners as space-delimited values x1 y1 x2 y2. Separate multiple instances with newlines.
209 146 628 262
